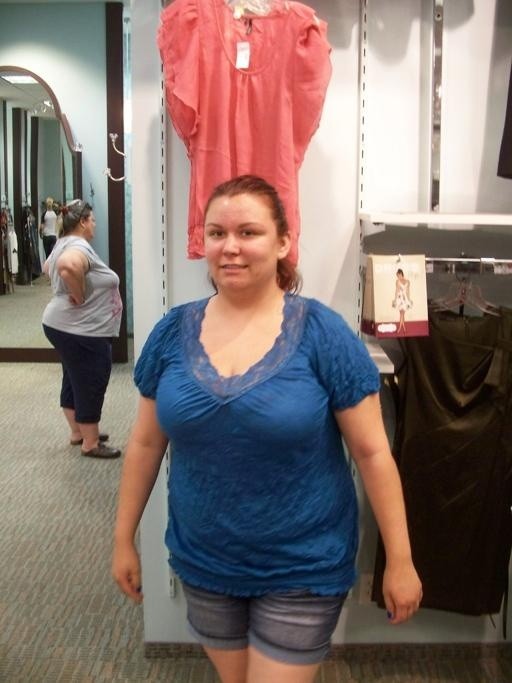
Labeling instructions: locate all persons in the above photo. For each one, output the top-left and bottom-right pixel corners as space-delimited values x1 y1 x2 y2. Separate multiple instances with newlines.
39 196 58 259
388 268 415 335
39 198 124 460
110 173 424 681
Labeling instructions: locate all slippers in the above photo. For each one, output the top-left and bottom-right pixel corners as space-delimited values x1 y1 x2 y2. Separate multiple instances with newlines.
80 442 121 459
70 432 109 446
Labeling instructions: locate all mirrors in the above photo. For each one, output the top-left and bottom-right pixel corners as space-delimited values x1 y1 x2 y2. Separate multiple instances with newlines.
0 66 82 363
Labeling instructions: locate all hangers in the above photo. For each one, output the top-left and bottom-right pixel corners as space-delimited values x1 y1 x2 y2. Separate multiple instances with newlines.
428 262 501 318
